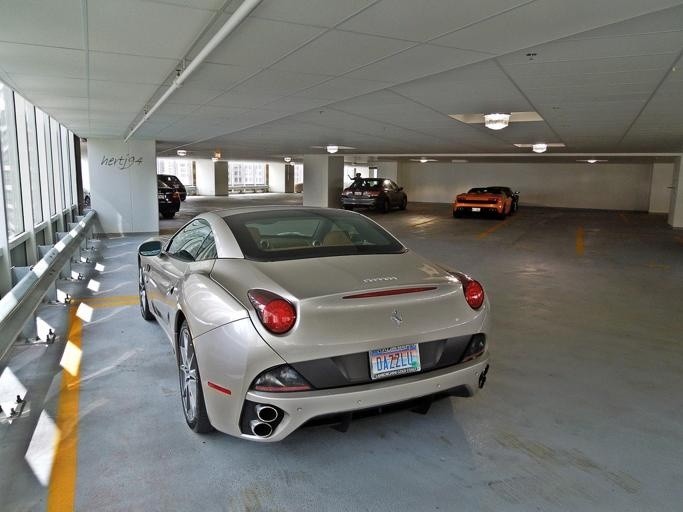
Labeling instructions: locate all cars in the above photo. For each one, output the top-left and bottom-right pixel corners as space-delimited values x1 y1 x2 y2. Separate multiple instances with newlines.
340 177 407 213
157 174 187 218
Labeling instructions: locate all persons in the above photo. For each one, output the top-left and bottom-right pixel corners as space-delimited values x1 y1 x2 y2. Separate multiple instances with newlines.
347 172 361 180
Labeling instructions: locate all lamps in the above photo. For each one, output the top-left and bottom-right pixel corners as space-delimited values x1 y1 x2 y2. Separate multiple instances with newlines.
176 144 224 163
531 143 546 156
483 111 512 132
282 143 342 163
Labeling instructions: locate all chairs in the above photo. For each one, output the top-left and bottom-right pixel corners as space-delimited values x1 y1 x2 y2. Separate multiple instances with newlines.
247 226 353 247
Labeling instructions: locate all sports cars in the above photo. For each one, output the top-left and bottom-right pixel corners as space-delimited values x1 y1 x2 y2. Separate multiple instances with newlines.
451 186 520 220
136 204 491 444
